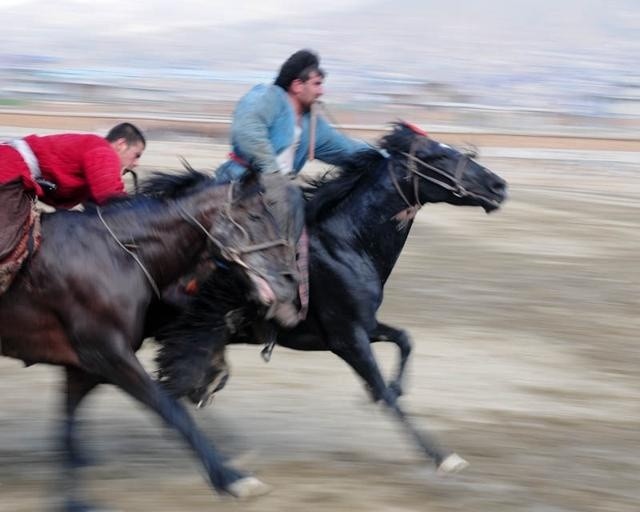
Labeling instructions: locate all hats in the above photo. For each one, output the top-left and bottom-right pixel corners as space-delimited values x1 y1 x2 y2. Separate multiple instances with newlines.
274 49 318 92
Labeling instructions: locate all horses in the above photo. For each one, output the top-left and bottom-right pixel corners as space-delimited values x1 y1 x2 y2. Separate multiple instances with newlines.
125 115 507 476
1 152 302 511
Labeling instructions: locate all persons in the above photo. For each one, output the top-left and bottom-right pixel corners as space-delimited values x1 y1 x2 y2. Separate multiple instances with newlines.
0 123 147 300
213 48 384 336
